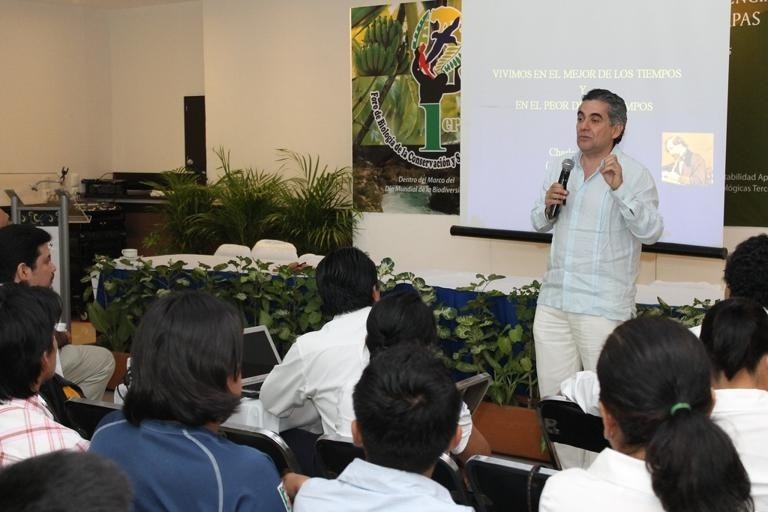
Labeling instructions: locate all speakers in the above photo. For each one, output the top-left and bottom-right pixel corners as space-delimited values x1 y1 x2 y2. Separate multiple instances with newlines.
184 95 206 170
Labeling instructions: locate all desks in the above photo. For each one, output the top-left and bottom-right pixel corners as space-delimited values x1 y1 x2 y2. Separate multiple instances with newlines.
633 278 727 340
470 401 551 461
221 396 320 434
380 264 539 376
89 239 326 351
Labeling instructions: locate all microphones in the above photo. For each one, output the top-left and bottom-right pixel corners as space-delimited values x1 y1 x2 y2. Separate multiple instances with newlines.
549 159 575 215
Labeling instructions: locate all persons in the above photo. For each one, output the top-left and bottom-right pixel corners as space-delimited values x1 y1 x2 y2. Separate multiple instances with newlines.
531 88 664 397
661 138 707 185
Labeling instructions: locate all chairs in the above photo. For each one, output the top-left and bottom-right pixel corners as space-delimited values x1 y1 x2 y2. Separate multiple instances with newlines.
314 435 364 478
64 398 123 441
219 424 301 478
434 454 560 512
105 348 132 392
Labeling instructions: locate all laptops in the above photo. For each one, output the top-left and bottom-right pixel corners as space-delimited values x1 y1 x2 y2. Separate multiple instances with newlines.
238 325 281 392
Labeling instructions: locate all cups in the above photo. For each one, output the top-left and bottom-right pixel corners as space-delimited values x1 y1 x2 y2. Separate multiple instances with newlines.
54 322 67 332
121 249 138 258
